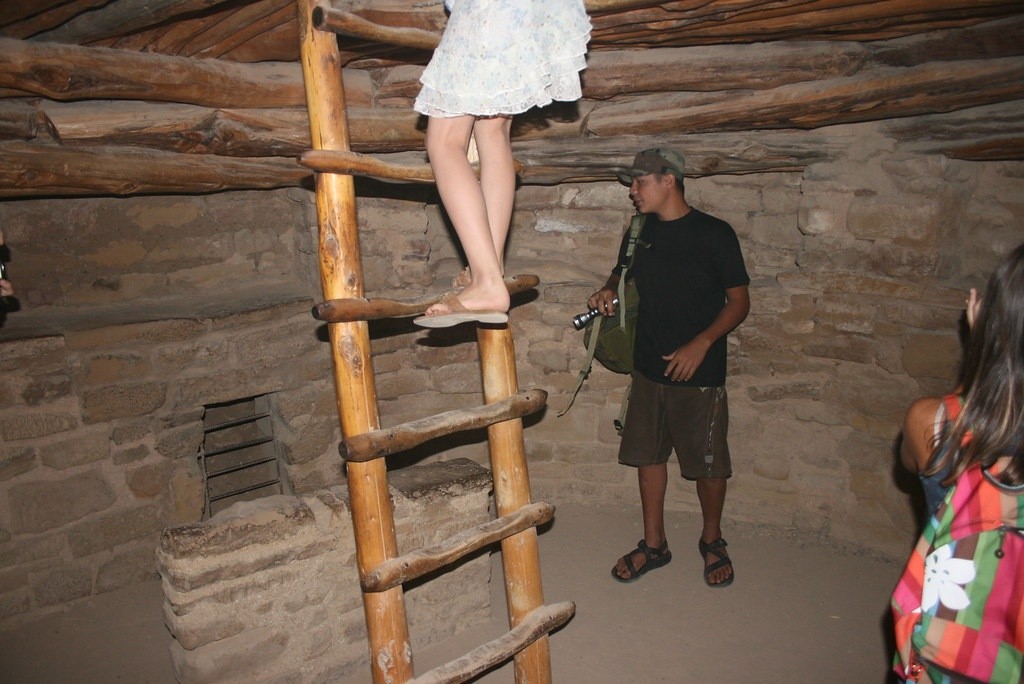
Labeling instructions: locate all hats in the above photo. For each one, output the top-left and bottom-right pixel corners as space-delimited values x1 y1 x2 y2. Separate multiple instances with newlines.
618 147 685 184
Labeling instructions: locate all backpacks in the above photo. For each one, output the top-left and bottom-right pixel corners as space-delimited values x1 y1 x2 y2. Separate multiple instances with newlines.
891 394 1024 683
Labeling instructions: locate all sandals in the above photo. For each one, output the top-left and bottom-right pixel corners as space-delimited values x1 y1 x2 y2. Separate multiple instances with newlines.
699 536 734 588
611 538 672 583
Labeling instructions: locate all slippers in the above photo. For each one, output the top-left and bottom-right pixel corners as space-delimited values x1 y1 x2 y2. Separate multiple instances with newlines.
413 291 509 328
452 268 540 294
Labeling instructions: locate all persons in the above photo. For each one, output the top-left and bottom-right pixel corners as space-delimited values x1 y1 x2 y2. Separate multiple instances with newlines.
587 148 752 586
888 241 1024 684
413 0 593 330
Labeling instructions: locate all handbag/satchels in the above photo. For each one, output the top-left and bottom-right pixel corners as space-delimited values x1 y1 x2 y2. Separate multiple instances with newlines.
582 214 646 374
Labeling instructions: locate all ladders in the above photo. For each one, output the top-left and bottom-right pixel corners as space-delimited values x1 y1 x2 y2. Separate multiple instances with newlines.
298 0 578 684
